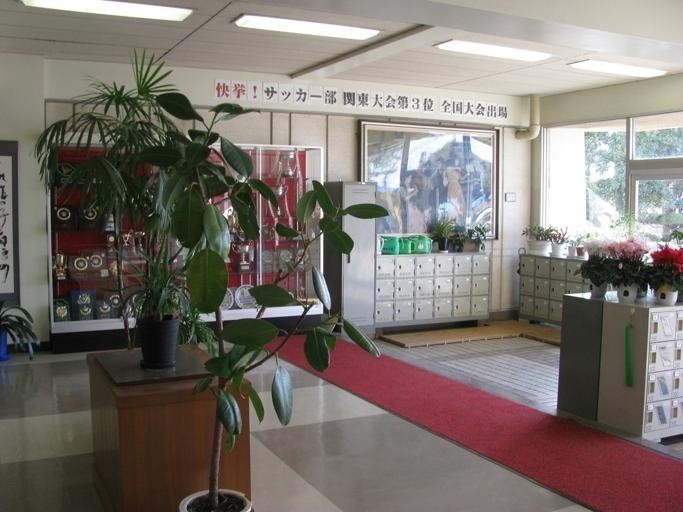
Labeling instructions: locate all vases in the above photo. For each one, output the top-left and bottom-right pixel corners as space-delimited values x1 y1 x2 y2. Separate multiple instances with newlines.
587 278 607 297
655 285 678 305
548 242 568 258
615 282 637 303
638 282 648 297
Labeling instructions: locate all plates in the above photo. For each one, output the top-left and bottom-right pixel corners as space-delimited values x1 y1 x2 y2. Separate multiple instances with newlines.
219 284 259 311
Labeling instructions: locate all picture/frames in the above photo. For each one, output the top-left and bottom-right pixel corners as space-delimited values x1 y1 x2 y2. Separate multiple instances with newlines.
353 118 500 242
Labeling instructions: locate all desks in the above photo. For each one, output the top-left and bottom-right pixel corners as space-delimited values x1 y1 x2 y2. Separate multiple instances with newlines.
80 342 256 512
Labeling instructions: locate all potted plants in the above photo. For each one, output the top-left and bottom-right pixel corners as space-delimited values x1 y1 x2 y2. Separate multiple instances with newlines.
28 43 282 371
426 214 457 251
566 239 577 257
521 223 558 255
0 297 43 363
448 223 490 252
99 87 396 512
575 234 591 258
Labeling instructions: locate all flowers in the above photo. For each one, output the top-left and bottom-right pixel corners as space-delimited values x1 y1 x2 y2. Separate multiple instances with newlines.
649 243 682 292
608 234 651 288
574 238 615 284
549 225 569 243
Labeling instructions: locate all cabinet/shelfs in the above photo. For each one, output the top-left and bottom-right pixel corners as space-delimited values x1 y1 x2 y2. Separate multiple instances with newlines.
554 292 603 416
516 253 593 326
322 181 376 340
596 300 683 439
375 252 492 332
44 134 328 354
